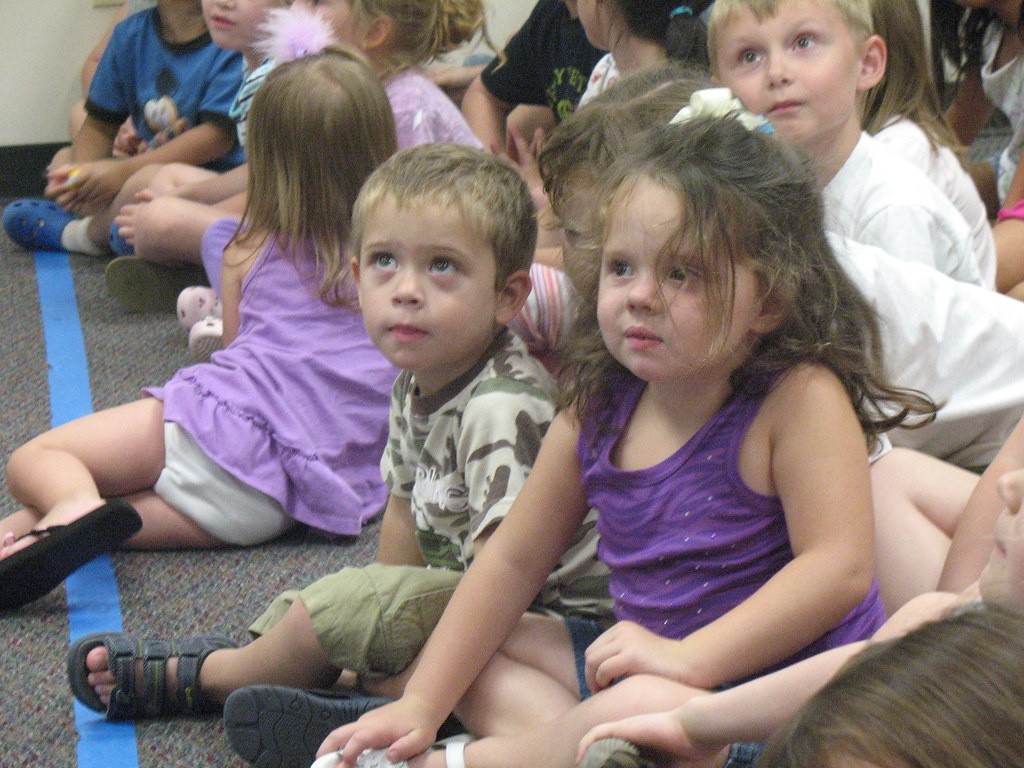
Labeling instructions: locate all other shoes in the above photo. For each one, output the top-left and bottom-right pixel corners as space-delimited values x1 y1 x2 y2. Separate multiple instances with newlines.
105 255 211 312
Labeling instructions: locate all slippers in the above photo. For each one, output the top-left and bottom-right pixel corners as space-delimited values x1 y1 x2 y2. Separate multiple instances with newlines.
0 501 143 611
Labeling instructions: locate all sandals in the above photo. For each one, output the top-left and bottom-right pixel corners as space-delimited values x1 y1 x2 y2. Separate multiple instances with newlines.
579 737 657 768
3 197 110 257
176 286 222 328
310 733 477 768
223 685 401 768
188 315 227 362
68 634 240 722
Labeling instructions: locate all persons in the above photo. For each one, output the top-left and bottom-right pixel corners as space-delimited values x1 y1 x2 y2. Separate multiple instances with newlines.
67 141 618 768
0 0 402 612
310 109 949 768
707 0 983 287
1 0 1023 768
